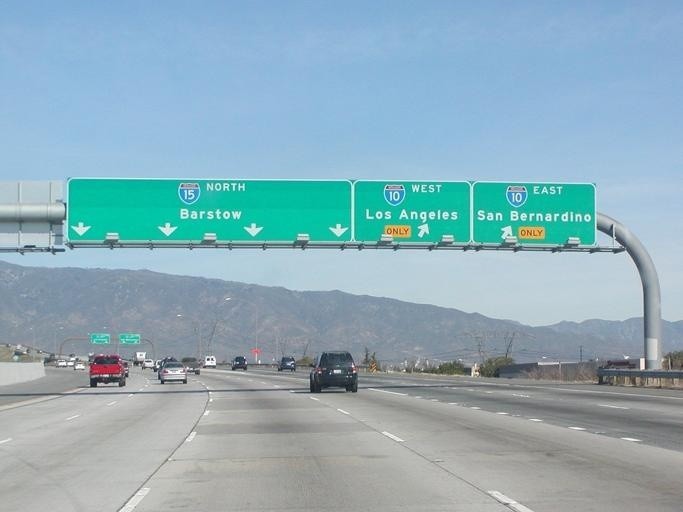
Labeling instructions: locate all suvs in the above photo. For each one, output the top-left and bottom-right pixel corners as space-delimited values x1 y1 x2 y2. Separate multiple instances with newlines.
87 351 130 390
307 350 361 393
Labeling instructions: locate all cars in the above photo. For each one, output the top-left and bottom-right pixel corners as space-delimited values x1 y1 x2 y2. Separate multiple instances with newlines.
140 356 201 385
230 356 249 371
276 357 297 371
4 342 85 373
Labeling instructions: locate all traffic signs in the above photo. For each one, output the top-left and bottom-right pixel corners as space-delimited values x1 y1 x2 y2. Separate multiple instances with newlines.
472 181 596 249
118 334 140 345
87 332 110 345
65 178 352 246
353 180 472 248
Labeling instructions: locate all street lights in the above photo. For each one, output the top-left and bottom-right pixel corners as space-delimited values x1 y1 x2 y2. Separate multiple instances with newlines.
176 314 203 359
224 296 260 364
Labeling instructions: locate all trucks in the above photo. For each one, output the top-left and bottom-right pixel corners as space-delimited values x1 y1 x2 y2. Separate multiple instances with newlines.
131 351 147 365
202 355 218 368
